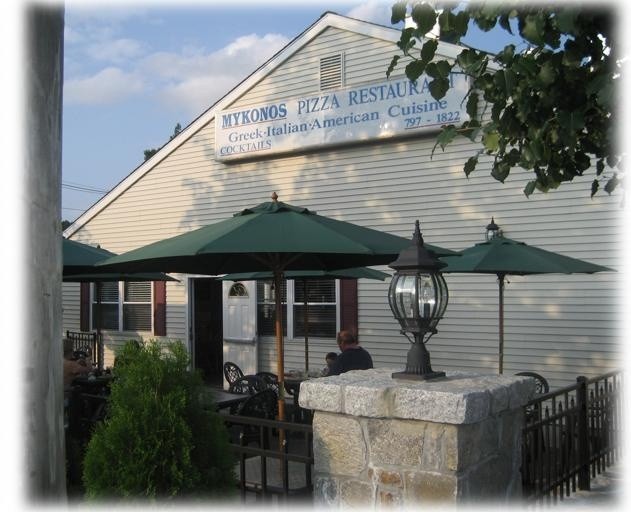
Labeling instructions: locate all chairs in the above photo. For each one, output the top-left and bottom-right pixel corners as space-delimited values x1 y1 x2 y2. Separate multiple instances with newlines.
64 344 550 511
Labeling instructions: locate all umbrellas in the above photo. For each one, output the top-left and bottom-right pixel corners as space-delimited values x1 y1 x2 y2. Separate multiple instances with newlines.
61 233 120 275
92 190 466 428
61 244 182 373
212 265 393 372
438 230 617 376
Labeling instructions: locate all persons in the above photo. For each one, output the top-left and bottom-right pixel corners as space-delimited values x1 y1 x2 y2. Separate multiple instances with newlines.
63 337 91 415
319 351 338 375
327 330 375 377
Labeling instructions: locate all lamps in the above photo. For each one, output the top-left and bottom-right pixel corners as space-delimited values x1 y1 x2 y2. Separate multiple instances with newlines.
387 221 449 381
486 216 502 244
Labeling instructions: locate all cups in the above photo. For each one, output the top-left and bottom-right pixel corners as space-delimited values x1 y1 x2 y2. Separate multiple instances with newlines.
309 368 320 377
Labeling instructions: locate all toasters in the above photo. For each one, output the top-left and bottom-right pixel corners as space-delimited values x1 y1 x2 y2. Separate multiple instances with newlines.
298 368 305 378
